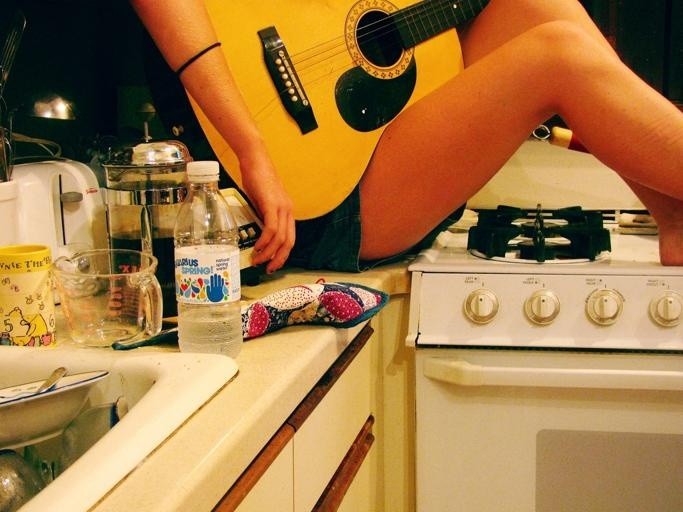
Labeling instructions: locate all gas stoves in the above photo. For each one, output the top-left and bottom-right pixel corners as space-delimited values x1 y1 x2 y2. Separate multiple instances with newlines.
408 204 679 350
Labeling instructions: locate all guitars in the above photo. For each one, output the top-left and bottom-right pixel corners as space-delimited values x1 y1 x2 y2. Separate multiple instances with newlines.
185 0 490 221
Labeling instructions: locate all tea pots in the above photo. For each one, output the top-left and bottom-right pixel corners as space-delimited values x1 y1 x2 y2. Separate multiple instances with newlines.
99 101 195 323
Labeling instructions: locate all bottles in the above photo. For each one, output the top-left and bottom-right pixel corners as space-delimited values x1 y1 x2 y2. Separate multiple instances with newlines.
173 161 243 359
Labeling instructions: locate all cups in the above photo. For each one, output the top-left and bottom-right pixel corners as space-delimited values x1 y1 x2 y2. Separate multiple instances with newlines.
55 248 162 347
0 402 113 511
0 244 56 349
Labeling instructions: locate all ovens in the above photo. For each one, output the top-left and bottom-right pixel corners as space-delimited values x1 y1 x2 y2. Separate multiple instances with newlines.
402 354 683 512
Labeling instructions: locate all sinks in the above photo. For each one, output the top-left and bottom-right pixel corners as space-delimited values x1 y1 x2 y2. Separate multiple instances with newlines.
0 343 240 512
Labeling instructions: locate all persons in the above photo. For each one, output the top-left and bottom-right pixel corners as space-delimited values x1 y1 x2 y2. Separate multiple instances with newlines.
121 0 683 271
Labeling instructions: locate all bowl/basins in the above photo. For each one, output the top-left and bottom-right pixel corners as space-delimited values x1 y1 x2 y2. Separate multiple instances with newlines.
0 369 108 452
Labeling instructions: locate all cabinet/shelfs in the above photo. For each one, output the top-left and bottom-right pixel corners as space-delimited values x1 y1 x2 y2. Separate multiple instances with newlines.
212 325 377 512
